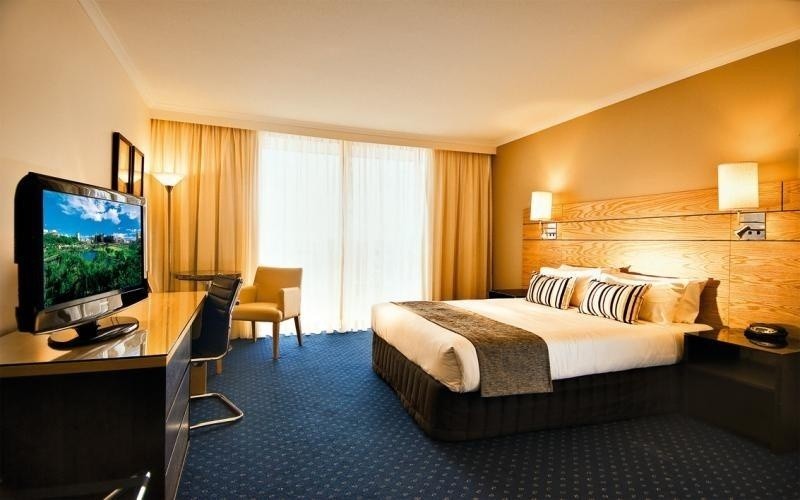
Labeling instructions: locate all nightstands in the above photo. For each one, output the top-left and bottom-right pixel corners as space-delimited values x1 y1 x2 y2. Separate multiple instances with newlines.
682 318 800 451
487 287 528 301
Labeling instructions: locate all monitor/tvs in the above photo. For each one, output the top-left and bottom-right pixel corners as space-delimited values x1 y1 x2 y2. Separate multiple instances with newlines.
14 172 147 349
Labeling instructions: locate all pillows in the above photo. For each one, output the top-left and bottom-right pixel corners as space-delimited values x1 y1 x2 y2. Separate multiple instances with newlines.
526 264 704 327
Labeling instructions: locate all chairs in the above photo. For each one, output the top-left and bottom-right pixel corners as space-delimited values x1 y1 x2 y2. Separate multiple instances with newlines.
189 272 248 437
230 264 307 363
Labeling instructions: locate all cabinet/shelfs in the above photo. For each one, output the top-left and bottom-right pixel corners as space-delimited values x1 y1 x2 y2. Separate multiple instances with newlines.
1 283 210 500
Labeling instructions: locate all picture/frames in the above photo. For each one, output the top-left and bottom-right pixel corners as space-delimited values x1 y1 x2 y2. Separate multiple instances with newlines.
110 131 133 194
129 144 146 198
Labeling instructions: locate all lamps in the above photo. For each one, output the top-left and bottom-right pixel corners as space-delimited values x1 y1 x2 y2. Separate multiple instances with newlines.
716 160 770 245
527 189 559 241
148 160 189 291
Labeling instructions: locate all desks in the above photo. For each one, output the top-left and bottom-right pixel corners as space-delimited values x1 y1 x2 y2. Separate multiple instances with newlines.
174 267 244 293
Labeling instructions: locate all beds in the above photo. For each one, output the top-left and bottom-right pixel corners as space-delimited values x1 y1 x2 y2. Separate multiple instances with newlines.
368 262 707 441
